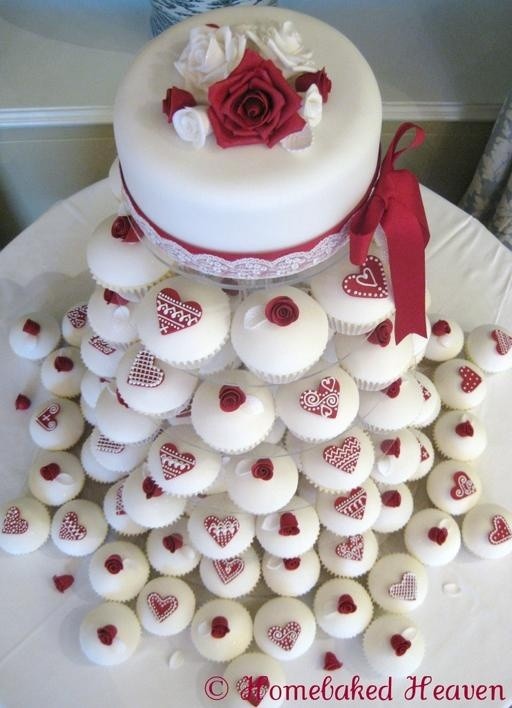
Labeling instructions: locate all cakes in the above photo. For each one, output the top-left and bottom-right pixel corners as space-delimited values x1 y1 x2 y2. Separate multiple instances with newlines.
2 6 512 708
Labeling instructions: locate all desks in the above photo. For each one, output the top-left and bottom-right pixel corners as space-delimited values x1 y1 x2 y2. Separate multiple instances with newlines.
2 2 512 134
1 175 510 706
